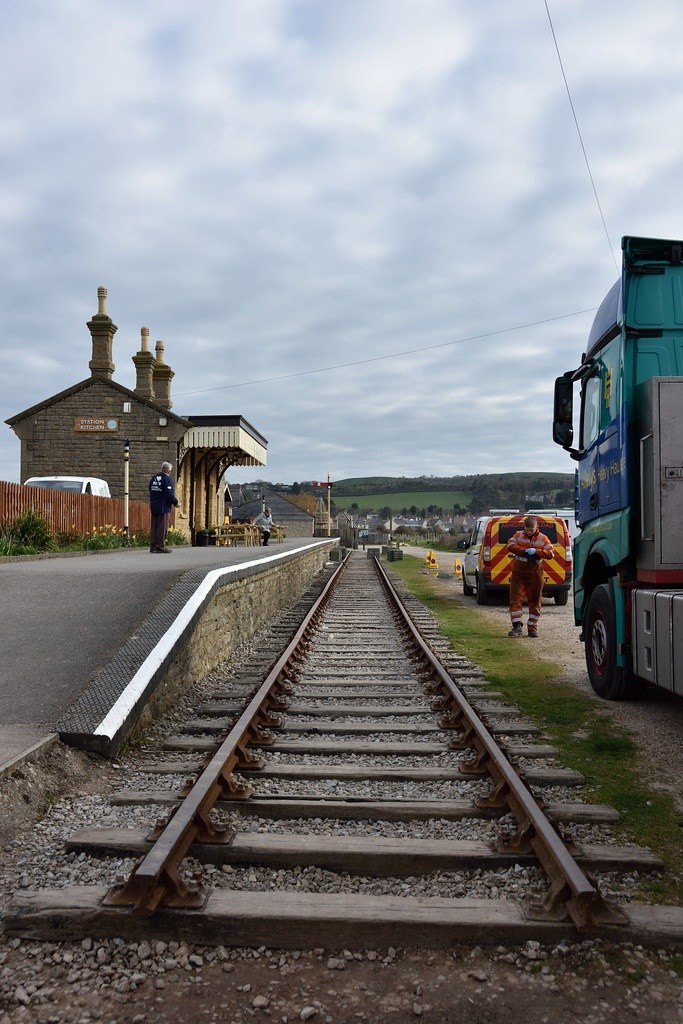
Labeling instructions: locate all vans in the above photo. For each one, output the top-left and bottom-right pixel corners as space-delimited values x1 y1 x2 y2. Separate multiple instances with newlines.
457 509 573 606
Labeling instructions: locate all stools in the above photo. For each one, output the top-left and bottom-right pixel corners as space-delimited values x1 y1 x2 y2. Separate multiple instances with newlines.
262 534 287 535
215 537 255 538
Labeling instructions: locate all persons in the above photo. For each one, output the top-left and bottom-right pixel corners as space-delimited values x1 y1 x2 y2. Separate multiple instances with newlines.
149 461 180 553
252 508 275 546
507 517 556 638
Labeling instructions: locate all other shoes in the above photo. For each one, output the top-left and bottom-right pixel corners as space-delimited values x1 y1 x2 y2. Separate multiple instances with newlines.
150 547 172 553
508 623 522 636
528 629 538 637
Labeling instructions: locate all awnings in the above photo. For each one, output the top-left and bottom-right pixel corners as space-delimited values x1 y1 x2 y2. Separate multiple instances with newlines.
181 414 268 466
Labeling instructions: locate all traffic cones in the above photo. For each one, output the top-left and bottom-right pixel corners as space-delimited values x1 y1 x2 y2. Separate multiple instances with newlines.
425 550 433 564
453 558 462 575
427 551 439 568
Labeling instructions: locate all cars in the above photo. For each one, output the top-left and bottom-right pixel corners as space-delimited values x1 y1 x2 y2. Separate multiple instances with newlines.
23 476 111 497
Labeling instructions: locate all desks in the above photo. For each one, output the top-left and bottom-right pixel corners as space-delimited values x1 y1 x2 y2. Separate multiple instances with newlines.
210 524 288 548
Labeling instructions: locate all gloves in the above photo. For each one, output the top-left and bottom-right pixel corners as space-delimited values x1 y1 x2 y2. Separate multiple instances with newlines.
525 548 536 555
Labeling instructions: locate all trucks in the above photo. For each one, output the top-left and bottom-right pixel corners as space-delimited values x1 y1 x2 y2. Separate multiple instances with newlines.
551 233 683 704
